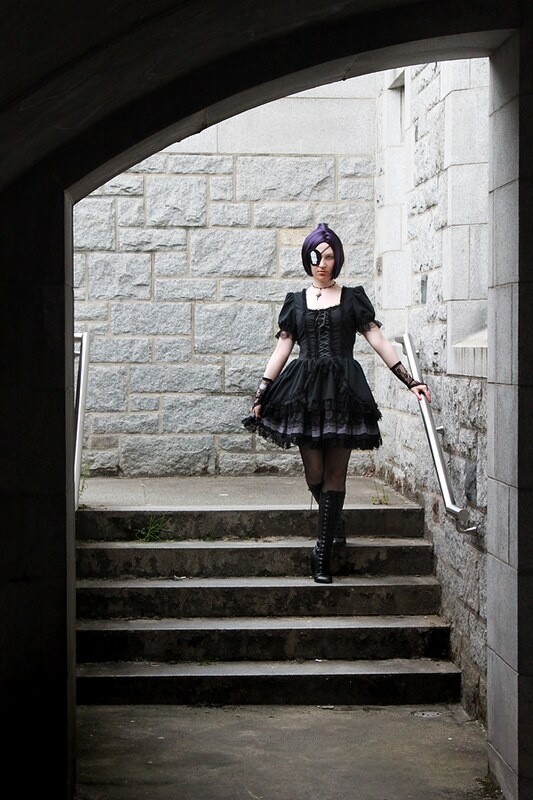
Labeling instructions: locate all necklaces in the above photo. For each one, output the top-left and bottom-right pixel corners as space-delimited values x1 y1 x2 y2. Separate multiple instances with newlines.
311 281 336 300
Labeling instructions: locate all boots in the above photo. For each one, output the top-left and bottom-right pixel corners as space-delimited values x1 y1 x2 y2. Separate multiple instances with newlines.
309 484 346 546
312 490 338 583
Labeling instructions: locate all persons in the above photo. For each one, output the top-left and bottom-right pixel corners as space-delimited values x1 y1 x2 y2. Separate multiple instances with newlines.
242 222 433 582
